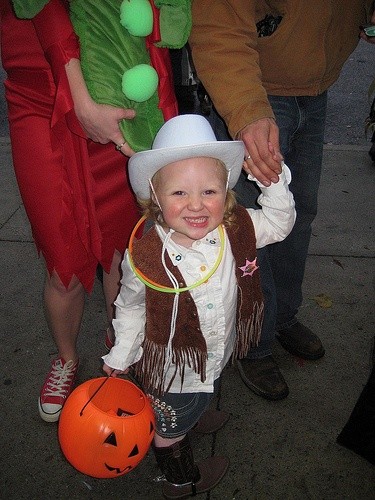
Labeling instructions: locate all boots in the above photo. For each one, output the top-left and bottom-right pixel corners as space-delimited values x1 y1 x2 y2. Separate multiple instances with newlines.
151 433 230 498
194 410 230 433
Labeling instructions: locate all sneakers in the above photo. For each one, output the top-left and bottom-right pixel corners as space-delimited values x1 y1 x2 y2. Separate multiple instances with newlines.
38 353 78 422
104 328 115 352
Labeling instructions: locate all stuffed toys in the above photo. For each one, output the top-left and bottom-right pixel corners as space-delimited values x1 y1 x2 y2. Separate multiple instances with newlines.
10 0 192 153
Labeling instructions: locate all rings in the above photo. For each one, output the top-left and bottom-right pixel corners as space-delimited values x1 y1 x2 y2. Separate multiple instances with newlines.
116 141 126 150
244 155 251 161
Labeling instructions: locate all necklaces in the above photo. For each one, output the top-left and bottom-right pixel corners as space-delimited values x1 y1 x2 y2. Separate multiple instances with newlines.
129 216 225 293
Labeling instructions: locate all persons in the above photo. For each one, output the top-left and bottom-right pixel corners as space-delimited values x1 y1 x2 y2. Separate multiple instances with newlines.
102 114 297 500
187 0 375 400
0 0 181 425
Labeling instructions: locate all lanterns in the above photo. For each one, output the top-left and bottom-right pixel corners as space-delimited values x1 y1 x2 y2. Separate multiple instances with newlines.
58 369 155 480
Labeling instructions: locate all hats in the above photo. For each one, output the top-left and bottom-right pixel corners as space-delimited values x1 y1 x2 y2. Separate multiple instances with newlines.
128 114 245 205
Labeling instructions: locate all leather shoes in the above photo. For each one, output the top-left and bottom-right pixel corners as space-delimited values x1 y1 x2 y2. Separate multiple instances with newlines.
274 322 325 360
235 353 290 399
